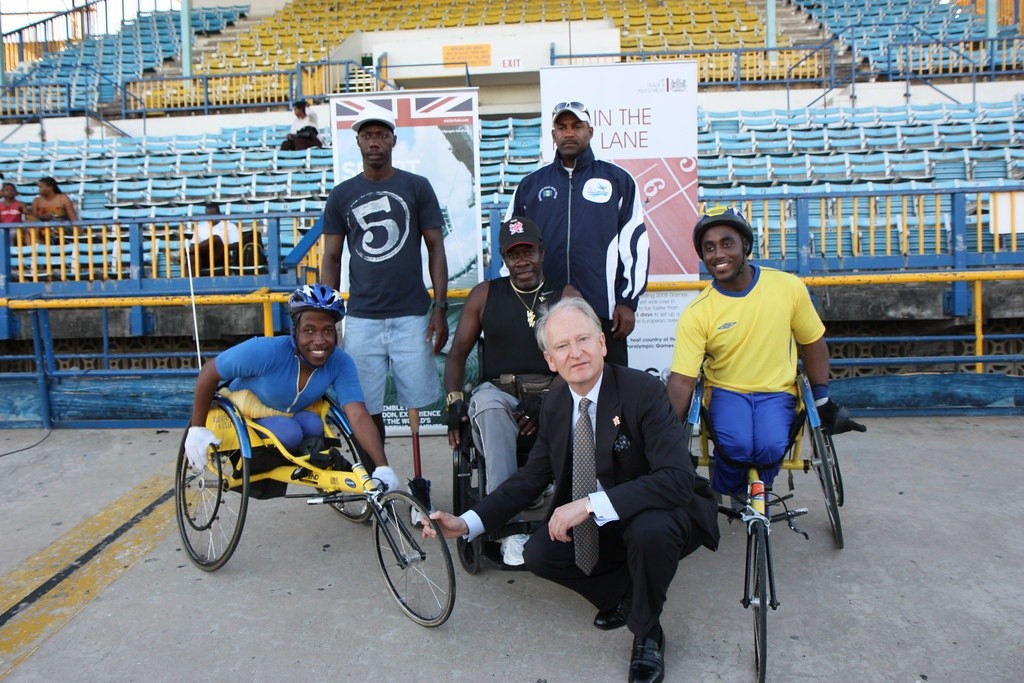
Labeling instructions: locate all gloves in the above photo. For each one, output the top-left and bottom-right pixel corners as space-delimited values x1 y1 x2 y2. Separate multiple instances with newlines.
184 426 223 471
817 398 868 436
372 464 400 505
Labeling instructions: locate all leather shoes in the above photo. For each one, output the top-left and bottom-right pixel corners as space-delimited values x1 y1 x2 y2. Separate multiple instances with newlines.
627 626 665 683
594 602 628 630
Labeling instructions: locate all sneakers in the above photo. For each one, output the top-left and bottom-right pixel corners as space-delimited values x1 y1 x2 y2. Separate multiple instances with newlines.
408 478 436 527
499 519 532 566
363 505 388 526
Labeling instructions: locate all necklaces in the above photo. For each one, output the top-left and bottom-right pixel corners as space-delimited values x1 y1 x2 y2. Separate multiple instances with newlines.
510 276 544 327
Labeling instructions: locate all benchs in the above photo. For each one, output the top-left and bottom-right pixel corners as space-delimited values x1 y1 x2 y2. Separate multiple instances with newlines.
0 0 1024 277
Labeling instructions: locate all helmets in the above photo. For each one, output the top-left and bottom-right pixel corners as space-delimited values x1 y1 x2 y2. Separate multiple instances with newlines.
692 206 753 261
289 284 345 321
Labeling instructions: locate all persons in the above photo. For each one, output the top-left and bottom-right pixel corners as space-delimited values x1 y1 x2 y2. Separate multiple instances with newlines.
320 106 448 528
498 100 650 367
421 296 720 683
163 202 239 266
25 176 83 245
280 98 322 151
667 206 839 495
0 183 29 223
445 216 583 511
185 283 400 495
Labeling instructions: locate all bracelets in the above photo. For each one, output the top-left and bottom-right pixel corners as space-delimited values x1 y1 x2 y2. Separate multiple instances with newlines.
576 499 595 516
446 391 464 406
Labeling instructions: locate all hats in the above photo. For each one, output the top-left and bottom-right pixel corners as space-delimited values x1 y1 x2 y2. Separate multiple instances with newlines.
551 100 593 130
350 106 395 133
498 216 543 254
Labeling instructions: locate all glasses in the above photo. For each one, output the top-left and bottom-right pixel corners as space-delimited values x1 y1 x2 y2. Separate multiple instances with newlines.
551 102 591 121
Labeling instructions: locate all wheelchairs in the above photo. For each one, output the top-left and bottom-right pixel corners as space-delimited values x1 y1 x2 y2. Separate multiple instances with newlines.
450 332 632 576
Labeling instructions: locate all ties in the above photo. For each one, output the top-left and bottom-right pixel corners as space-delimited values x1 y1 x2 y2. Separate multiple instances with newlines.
571 398 601 575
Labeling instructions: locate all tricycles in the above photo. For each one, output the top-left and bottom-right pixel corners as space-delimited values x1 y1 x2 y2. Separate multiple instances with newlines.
681 370 846 683
174 389 459 633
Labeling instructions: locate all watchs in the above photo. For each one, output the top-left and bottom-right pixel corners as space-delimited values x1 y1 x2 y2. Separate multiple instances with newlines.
432 300 449 312
